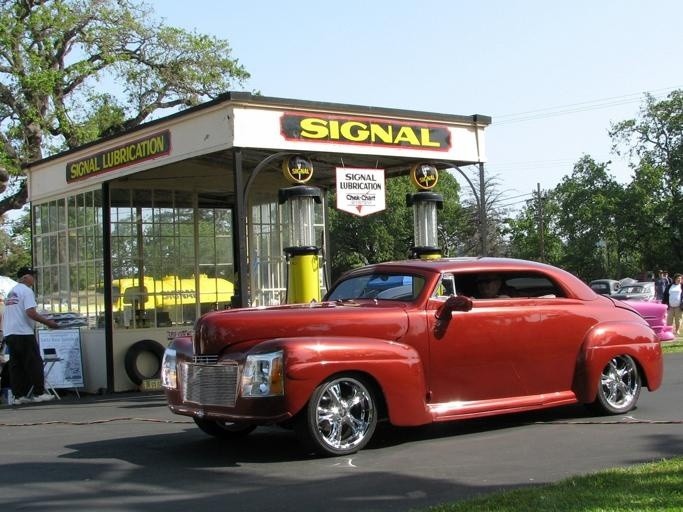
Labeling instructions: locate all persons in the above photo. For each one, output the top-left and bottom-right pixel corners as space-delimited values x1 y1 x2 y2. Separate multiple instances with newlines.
468 274 511 299
653 270 683 334
3 266 59 405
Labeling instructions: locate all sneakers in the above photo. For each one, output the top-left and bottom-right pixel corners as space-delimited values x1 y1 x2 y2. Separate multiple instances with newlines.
31 393 55 402
13 395 32 406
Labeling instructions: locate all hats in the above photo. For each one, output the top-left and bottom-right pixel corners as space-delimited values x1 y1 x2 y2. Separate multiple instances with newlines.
16 267 38 279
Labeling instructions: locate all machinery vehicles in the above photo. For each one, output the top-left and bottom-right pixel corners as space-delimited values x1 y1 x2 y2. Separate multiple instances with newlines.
85 274 235 315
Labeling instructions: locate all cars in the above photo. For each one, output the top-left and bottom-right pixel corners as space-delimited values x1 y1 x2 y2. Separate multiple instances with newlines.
589 275 674 341
159 256 664 457
